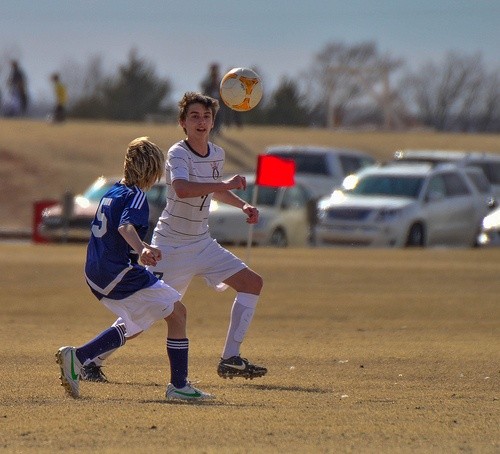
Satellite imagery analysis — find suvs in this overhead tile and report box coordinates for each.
[311,161,494,247]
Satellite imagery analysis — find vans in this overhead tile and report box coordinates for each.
[395,149,500,184]
[260,146,376,201]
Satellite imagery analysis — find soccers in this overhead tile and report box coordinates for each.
[220,67,263,112]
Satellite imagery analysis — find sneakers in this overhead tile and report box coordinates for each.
[216,353,268,381]
[79,361,110,383]
[165,380,215,400]
[54,346,84,399]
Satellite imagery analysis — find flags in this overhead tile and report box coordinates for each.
[255,154,296,187]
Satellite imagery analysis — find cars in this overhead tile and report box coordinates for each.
[207,175,311,248]
[476,197,499,246]
[36,176,169,244]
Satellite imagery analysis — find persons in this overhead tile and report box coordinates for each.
[8,58,66,124]
[54,91,268,402]
